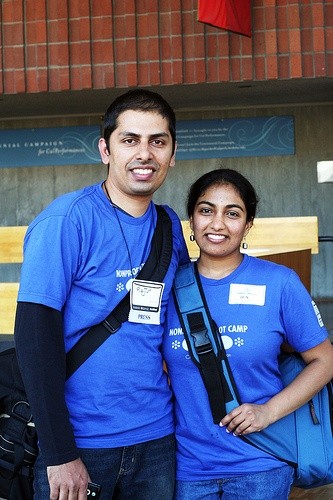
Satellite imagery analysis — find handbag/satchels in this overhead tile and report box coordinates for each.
[276,352,332,487]
[0,347,38,500]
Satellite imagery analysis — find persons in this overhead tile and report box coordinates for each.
[157,167,333,500]
[13,88,193,500]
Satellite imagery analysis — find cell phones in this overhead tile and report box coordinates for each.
[86,482,100,500]
